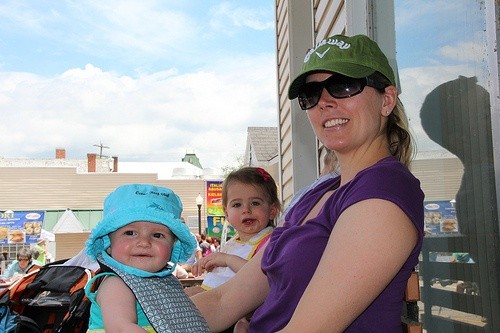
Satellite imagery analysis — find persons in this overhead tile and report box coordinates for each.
[183,147,341,333]
[187,34,426,333]
[181,217,220,267]
[7,248,39,278]
[30,248,45,266]
[85,184,211,333]
[191,166,283,290]
[35,238,47,264]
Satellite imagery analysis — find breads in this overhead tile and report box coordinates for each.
[0,222,24,242]
[442,219,456,231]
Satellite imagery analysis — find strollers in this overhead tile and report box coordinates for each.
[0,257,91,333]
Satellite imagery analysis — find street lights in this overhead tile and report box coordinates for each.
[196,193,204,235]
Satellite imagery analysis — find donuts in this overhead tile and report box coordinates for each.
[25,222,41,234]
[425,212,441,224]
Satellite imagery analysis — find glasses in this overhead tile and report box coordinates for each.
[297,73,383,111]
[17,258,29,262]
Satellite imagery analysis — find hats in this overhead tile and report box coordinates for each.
[85,184,197,278]
[287,34,396,101]
